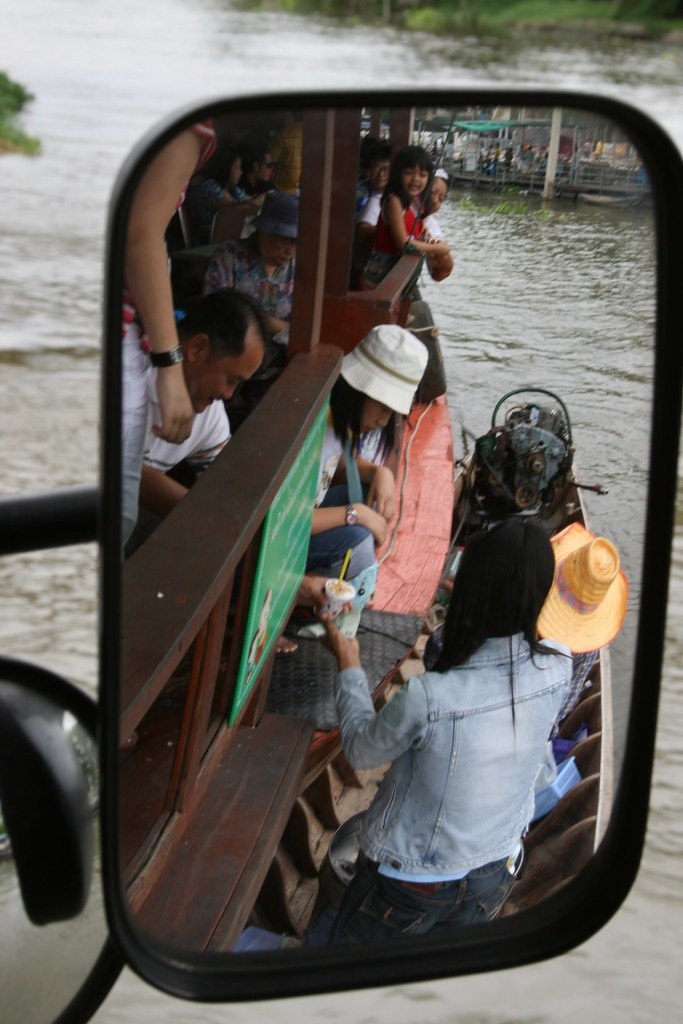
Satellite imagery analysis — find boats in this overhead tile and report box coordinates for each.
[234,389,618,953]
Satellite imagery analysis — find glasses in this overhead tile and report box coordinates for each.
[262,161,278,169]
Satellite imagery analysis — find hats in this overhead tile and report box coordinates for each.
[248,191,301,239]
[534,522,630,656]
[339,324,429,415]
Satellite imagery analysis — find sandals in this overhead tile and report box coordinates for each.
[276,639,299,658]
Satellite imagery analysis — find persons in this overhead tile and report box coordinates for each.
[121,102,626,954]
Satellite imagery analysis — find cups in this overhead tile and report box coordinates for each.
[314,578,356,622]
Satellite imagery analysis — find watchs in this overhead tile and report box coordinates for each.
[151,345,184,368]
[345,504,359,526]
